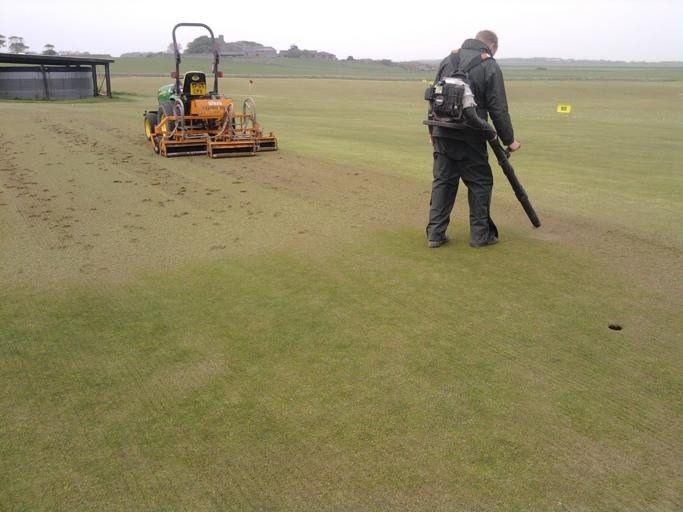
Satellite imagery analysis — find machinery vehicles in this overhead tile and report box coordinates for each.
[144,22,279,159]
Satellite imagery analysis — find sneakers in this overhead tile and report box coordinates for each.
[468,236,499,249]
[427,233,451,249]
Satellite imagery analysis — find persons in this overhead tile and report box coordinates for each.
[424,28,521,249]
